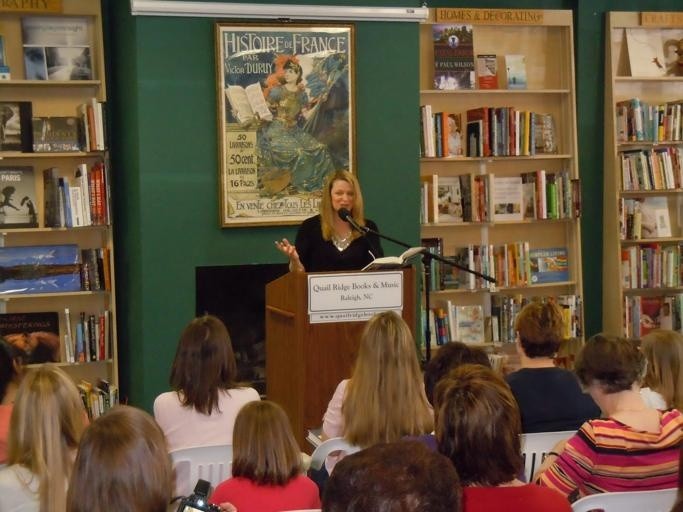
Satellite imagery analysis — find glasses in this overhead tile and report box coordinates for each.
[129,0,429,23]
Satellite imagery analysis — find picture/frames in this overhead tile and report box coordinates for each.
[264,264,417,447]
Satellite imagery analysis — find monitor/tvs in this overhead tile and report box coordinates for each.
[622,293,683,337]
[225,82,274,127]
[361,246,426,272]
[64,308,109,363]
[0,161,107,229]
[620,194,643,243]
[621,243,683,289]
[615,98,683,143]
[557,295,583,338]
[420,169,582,225]
[80,247,110,293]
[424,295,522,346]
[420,103,556,157]
[421,237,568,292]
[0,98,105,153]
[75,377,118,421]
[619,147,683,191]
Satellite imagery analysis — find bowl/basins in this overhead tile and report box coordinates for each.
[331,231,353,253]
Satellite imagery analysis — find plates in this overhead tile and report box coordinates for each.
[419,7,586,353]
[602,10,683,339]
[1,0,120,418]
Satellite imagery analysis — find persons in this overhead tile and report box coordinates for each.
[639,328,683,411]
[0,363,90,512]
[230,55,337,199]
[153,314,261,503]
[207,400,322,512]
[531,331,683,512]
[503,301,601,434]
[321,312,435,477]
[0,335,24,463]
[276,168,384,273]
[321,442,462,512]
[66,403,174,512]
[424,342,492,409]
[433,364,574,512]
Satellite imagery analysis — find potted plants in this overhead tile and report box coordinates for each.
[213,21,359,230]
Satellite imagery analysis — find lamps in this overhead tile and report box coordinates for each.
[569,487,682,511]
[308,437,361,477]
[518,429,580,485]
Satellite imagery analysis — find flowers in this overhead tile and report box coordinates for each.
[337,207,364,235]
